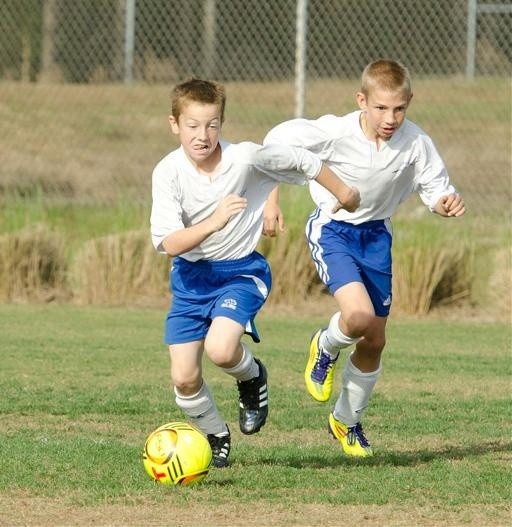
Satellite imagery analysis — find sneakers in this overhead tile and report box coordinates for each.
[328,411,373,458]
[207,425,230,469]
[237,357,268,436]
[305,327,340,403]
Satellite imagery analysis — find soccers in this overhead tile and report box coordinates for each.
[143,422,212,486]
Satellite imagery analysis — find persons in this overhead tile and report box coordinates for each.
[150,76,361,467]
[262,60,467,457]
